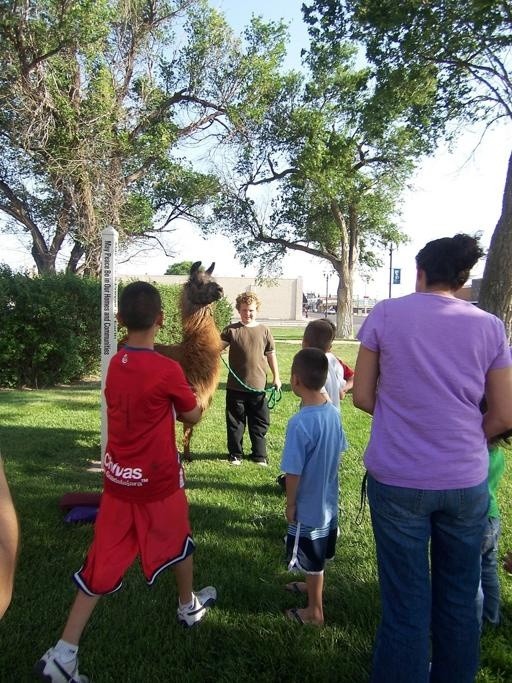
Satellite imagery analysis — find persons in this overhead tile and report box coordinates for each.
[35,282,217,683]
[0,457,20,620]
[219,291,282,468]
[280,319,353,626]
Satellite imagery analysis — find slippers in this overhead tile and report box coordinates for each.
[283,581,303,595]
[284,607,309,626]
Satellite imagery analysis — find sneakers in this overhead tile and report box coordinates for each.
[228,456,267,466]
[176,586,217,628]
[37,647,89,683]
